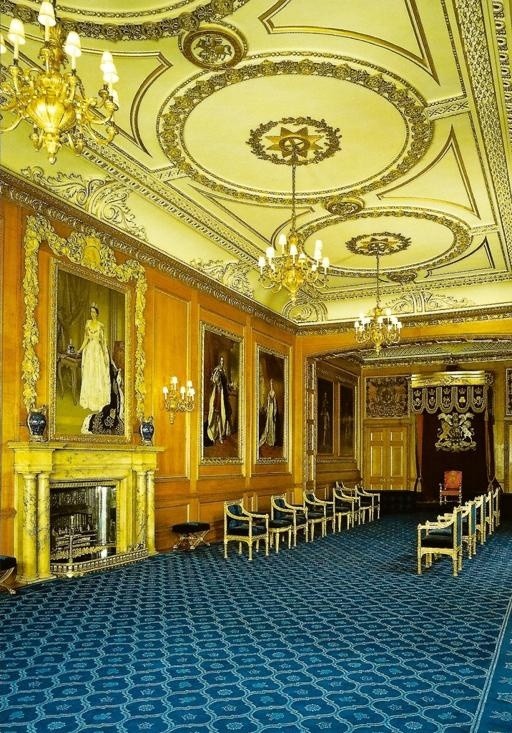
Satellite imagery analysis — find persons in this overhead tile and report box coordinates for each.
[77,302,118,412]
[260,378,279,448]
[206,354,237,444]
[320,391,332,447]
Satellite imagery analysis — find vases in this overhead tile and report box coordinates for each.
[25,408,47,442]
[139,421,154,446]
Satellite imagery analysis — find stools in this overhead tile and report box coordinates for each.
[173,522,211,550]
[0,555,17,595]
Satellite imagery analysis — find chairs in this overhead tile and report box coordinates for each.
[224,480,382,562]
[415,485,501,576]
[439,470,463,505]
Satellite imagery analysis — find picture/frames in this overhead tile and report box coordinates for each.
[505,368,512,415]
[316,376,356,457]
[254,341,289,465]
[48,256,133,444]
[197,320,248,465]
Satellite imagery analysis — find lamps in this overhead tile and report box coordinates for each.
[259,125,330,302]
[163,376,196,424]
[0,0,119,164]
[354,238,402,352]
